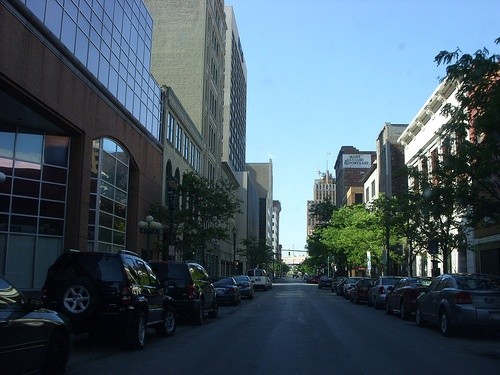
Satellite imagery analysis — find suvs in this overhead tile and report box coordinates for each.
[40,249,176,349]
[148,260,218,325]
[246,269,269,291]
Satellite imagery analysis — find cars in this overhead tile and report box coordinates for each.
[349,278,378,304]
[318,275,332,289]
[415,273,500,337]
[293,272,299,278]
[233,275,255,299]
[367,276,404,309]
[307,275,318,283]
[267,277,273,288]
[212,277,242,306]
[0,276,70,375]
[329,277,365,298]
[385,277,432,321]
